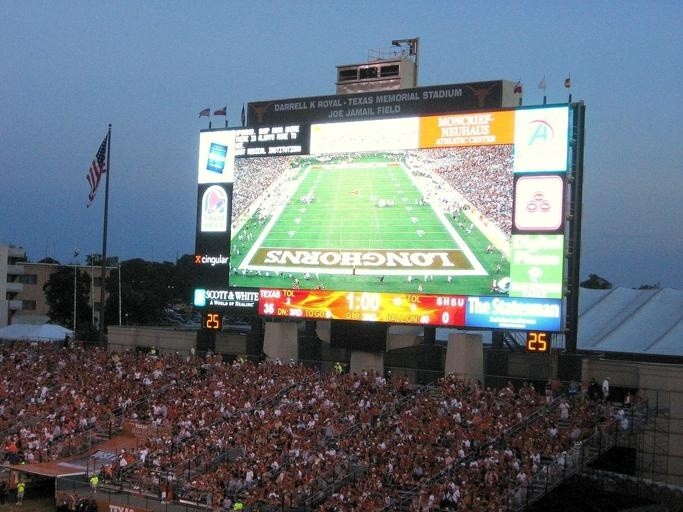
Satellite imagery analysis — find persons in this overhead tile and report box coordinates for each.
[229,144,514,296]
[1,340,632,512]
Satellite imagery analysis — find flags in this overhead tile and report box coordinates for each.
[565,78,570,88]
[213,107,226,115]
[539,79,544,89]
[85,129,109,210]
[513,83,523,94]
[240,106,245,125]
[198,109,210,118]
